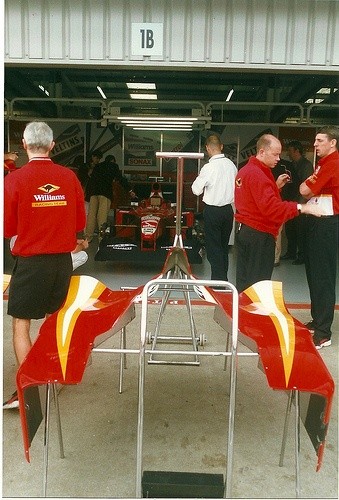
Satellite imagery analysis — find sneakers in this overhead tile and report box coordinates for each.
[312,336,332,349]
[304,320,316,334]
[3,391,20,409]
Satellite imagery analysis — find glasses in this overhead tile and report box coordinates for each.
[281,165,293,183]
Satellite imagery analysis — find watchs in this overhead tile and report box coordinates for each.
[297,204,302,214]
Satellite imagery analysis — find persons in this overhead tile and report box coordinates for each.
[76,150,103,236]
[86,155,135,242]
[299,128,339,350]
[3,121,86,410]
[191,135,238,290]
[234,134,320,295]
[272,141,314,267]
[4,141,88,271]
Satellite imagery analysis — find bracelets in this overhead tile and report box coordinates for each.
[129,190,132,192]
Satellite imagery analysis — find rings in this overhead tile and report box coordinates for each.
[282,176,285,179]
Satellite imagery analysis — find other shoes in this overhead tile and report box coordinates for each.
[281,254,295,260]
[292,260,304,265]
